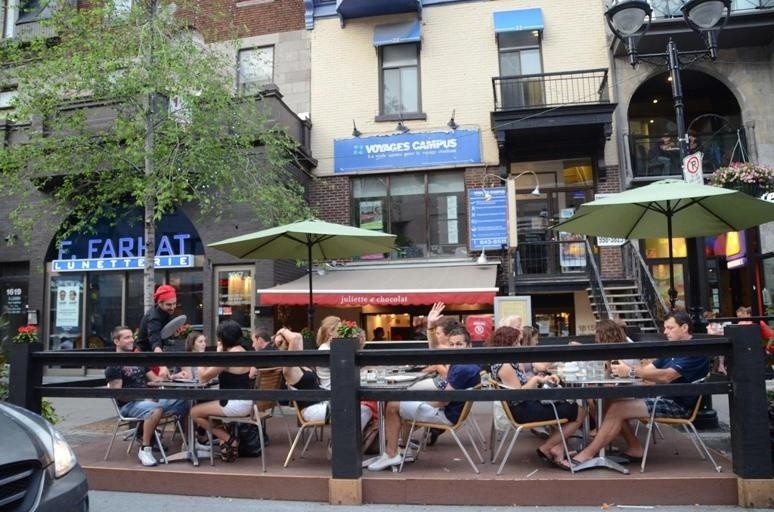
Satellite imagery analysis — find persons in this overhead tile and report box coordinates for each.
[646,130,705,175]
[704,307,774,375]
[105,287,274,471]
[489,310,712,471]
[275,298,481,471]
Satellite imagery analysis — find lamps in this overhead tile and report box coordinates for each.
[446,109,457,129]
[352,120,362,138]
[396,114,407,132]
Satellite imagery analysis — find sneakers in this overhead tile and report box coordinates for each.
[362,436,419,471]
[136,437,168,466]
[531,427,549,440]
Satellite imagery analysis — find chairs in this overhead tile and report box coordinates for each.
[103,383,189,466]
[206,366,293,472]
[488,376,570,475]
[395,384,487,474]
[633,371,722,474]
[283,382,331,468]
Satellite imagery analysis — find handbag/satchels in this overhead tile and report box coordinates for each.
[240,425,261,457]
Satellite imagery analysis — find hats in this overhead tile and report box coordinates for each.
[153,286,176,304]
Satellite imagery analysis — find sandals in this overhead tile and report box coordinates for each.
[219,435,239,463]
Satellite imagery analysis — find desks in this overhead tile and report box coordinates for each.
[558,371,643,476]
[147,379,219,467]
[359,365,430,472]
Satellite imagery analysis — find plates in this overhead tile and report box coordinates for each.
[159,314,187,342]
[386,371,426,381]
[173,378,198,384]
[561,365,581,373]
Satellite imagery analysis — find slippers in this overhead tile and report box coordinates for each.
[537,445,647,471]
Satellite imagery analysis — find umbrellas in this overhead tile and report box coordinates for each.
[205,219,397,329]
[546,179,774,310]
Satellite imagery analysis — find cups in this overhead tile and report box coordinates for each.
[360,365,404,385]
[585,359,605,381]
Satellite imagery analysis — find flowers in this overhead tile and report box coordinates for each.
[11,325,40,344]
[177,324,192,338]
[708,162,773,201]
[337,318,360,339]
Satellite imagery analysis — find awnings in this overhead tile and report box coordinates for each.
[257,287,499,305]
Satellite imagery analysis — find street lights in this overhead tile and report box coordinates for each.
[601,0,735,433]
[480,168,541,297]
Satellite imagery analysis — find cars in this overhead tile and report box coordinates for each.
[0,399,92,512]
